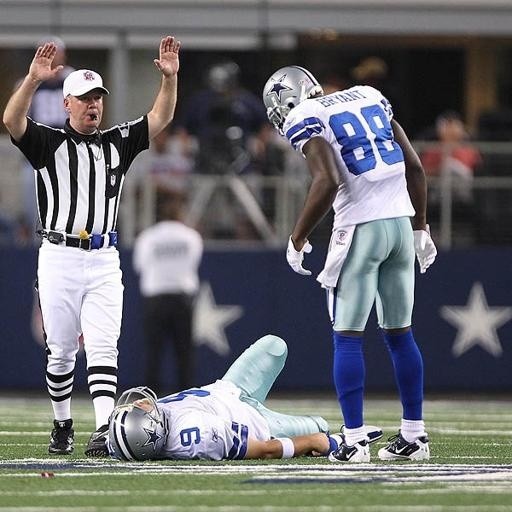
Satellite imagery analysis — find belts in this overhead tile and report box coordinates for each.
[42,230,117,252]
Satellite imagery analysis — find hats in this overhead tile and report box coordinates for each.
[63,69,110,99]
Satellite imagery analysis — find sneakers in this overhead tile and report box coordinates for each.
[326,436,370,463]
[47,418,74,455]
[377,428,431,461]
[84,424,109,459]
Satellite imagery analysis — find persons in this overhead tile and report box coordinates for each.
[263,65,439,463]
[2,35,181,459]
[133,190,203,397]
[0,29,511,244]
[108,334,383,461]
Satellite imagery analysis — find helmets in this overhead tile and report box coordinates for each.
[108,385,169,461]
[262,65,324,137]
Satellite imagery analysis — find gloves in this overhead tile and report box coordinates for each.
[285,234,314,277]
[413,222,438,274]
